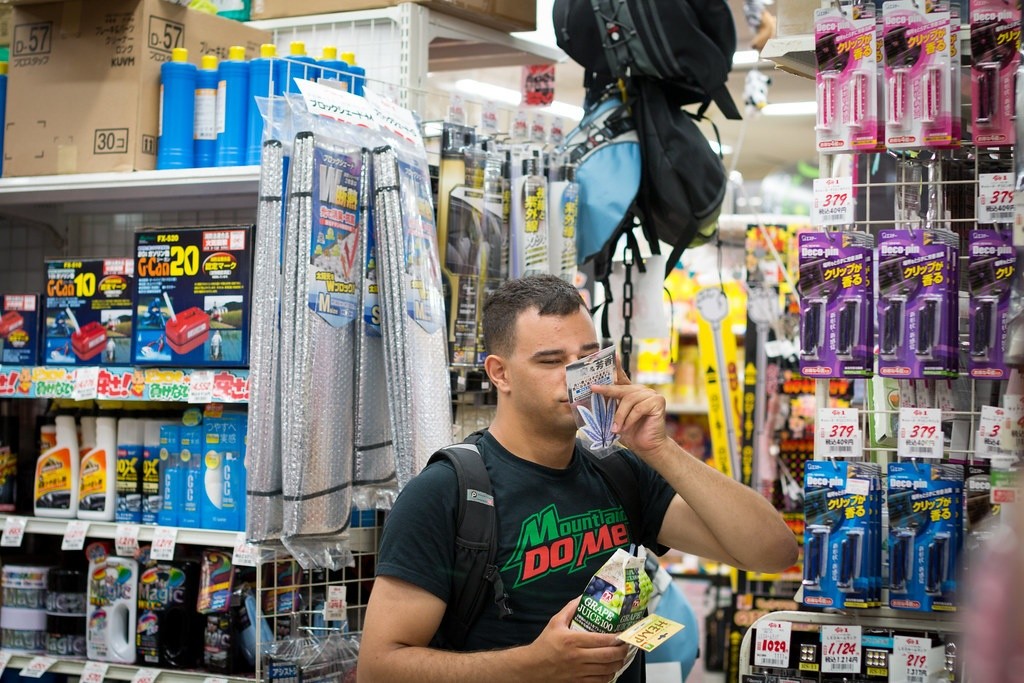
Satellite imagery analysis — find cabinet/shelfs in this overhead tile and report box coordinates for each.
[0,0,568,683]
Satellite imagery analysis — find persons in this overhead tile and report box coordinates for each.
[356,274,799,683]
[54,311,115,361]
[147,297,223,359]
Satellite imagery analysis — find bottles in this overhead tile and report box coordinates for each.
[32,398,180,525]
[157,39,364,170]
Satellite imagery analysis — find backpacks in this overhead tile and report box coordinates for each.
[553,0,744,279]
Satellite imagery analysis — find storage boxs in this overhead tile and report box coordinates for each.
[176,425,202,532]
[0,0,275,179]
[156,424,179,529]
[41,255,138,369]
[201,408,247,533]
[0,292,40,364]
[132,218,257,372]
